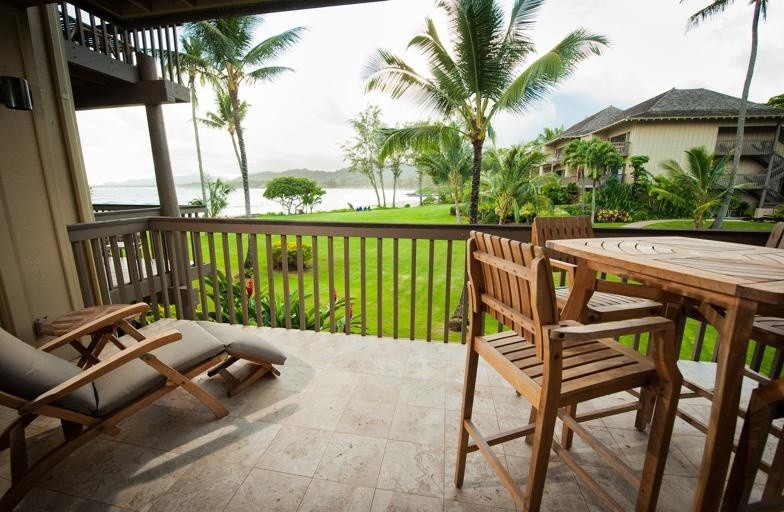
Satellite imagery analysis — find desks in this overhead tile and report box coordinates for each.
[521,233,784,511]
[42,303,139,371]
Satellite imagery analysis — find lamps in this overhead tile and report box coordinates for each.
[0,74,34,113]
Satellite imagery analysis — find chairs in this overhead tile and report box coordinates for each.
[455,228,685,512]
[719,378,784,511]
[1,301,286,512]
[658,220,784,499]
[513,214,665,454]
